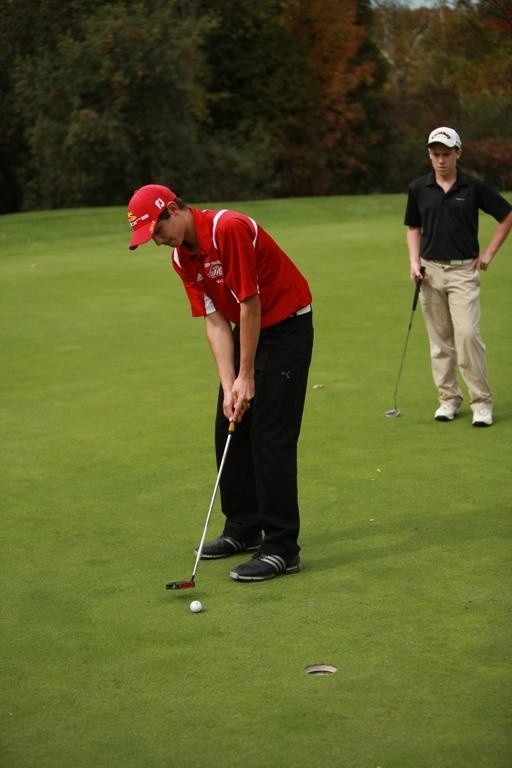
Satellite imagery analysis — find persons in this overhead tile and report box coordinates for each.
[122,182,313,584]
[401,123,512,429]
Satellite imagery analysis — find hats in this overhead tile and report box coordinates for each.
[128,184,176,250]
[427,127,462,149]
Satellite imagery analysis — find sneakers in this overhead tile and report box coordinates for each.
[229,552,300,581]
[434,404,460,420]
[473,405,493,426]
[194,533,263,558]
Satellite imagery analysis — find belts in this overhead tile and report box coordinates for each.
[434,259,474,265]
[289,304,311,317]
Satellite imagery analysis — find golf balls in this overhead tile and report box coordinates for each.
[190,601,202,611]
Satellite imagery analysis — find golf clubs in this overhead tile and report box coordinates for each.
[384,267,426,418]
[165,422,238,590]
